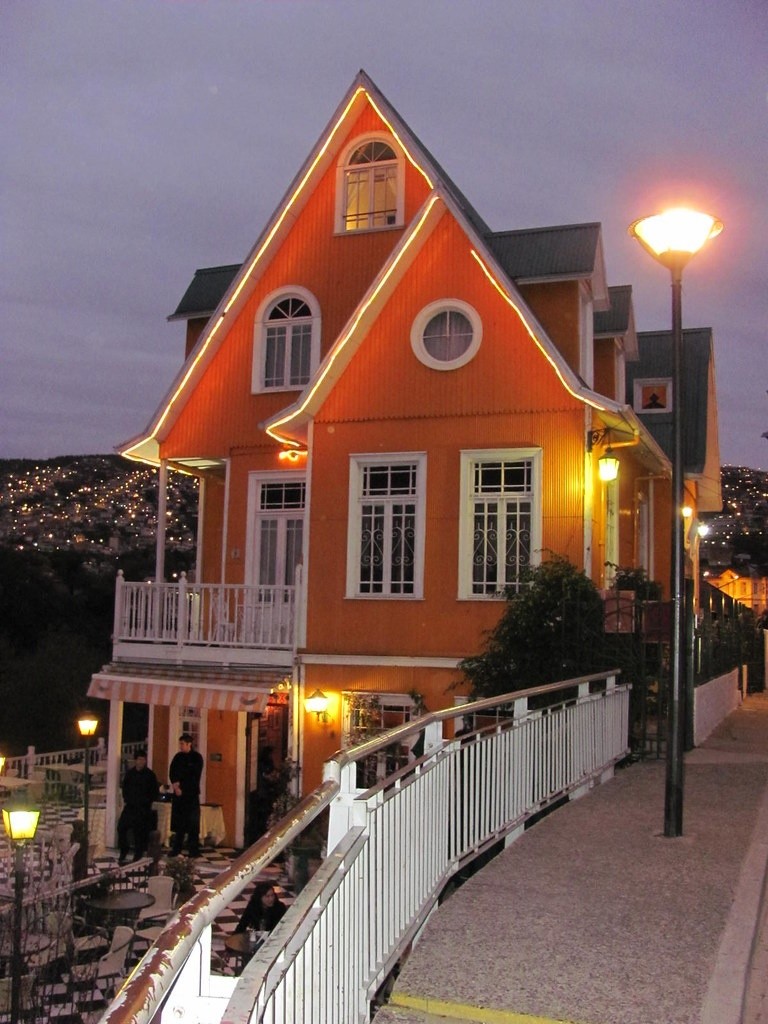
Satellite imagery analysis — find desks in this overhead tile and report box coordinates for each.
[235,602,286,645]
[146,801,226,850]
[85,891,155,927]
[225,931,268,956]
[0,932,52,968]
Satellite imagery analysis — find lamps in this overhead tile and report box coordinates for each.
[587,426,621,481]
[303,689,329,721]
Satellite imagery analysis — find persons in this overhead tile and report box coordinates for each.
[116,750,159,862]
[233,883,287,935]
[167,734,204,858]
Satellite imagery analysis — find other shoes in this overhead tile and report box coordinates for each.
[168,848,181,856]
[119,848,128,861]
[134,850,142,861]
[189,848,198,857]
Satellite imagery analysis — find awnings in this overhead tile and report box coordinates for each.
[87,664,292,714]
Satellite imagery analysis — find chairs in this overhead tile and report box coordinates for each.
[0,757,175,1024]
[210,594,237,647]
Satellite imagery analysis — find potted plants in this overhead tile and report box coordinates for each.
[269,791,318,893]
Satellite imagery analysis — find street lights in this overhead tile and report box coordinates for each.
[76,709,99,842]
[623,206,726,836]
[1,799,41,1024]
